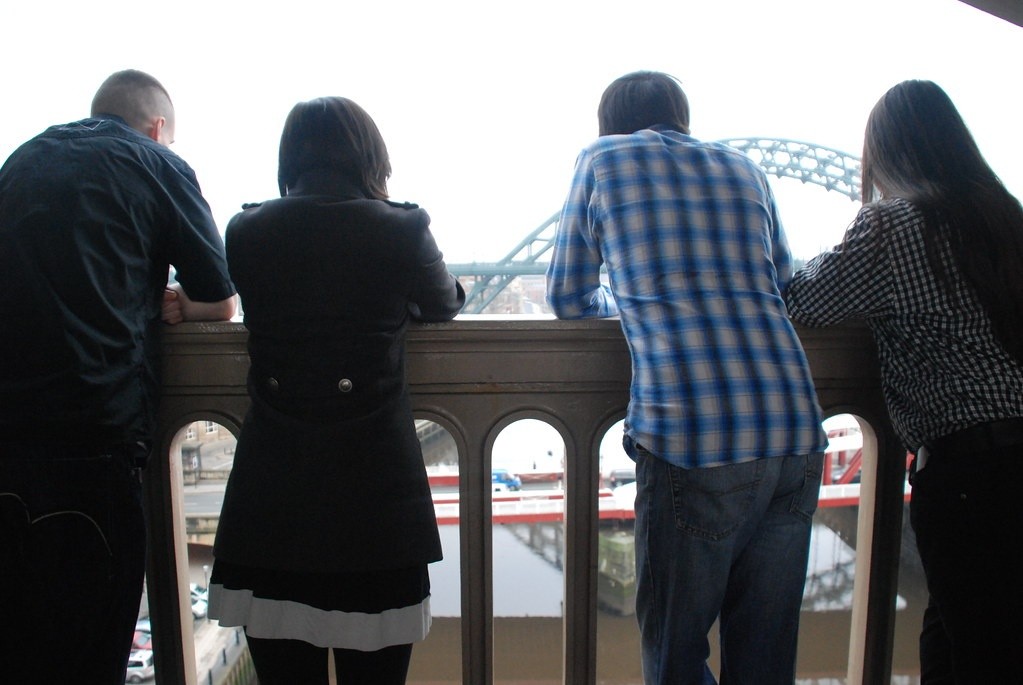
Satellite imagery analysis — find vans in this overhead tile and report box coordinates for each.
[491,469,522,490]
[121,649,157,683]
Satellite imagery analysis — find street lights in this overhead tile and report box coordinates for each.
[203,564,211,623]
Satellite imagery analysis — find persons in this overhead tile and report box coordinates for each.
[192,97,468,685]
[0,68,238,685]
[545,67,832,685]
[772,78,1023,685]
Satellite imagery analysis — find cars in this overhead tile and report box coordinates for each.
[188,582,210,602]
[828,466,862,487]
[187,596,208,621]
[491,482,511,494]
[130,631,154,652]
[135,615,156,632]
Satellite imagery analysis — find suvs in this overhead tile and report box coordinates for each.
[609,468,637,487]
